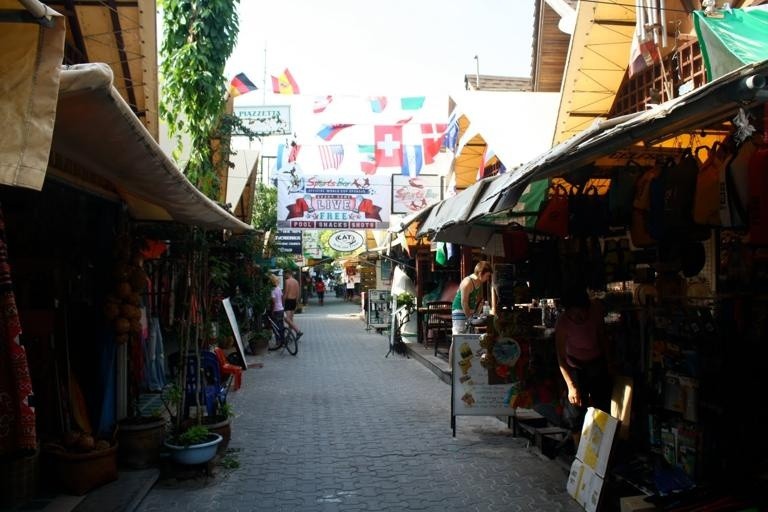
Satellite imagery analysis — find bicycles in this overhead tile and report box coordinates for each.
[254,307,299,357]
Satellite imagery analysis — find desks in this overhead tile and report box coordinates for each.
[433,315,452,357]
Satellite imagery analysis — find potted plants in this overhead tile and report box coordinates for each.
[249,327,274,356]
[159,224,250,457]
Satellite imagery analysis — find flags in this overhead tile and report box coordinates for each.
[231,67,448,179]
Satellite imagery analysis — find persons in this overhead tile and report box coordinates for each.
[447,260,492,374]
[305,273,356,306]
[554,289,603,451]
[281,269,303,341]
[271,277,284,349]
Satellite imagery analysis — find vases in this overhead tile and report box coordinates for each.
[113,415,168,470]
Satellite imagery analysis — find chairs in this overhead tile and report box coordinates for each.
[419,300,452,350]
